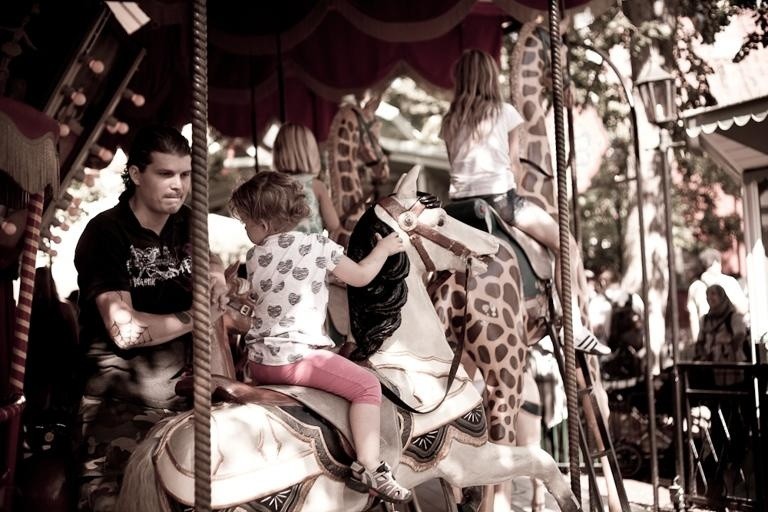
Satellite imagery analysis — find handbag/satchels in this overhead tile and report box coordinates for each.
[444,198,498,234]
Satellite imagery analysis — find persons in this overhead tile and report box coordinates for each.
[585,248,747,498]
[22,49,610,512]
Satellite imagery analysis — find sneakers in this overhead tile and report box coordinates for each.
[559,325,613,355]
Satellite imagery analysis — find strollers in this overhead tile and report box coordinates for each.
[613,372,679,478]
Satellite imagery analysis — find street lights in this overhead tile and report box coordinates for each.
[635,55,686,512]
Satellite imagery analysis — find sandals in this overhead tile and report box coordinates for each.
[344,460,414,505]
